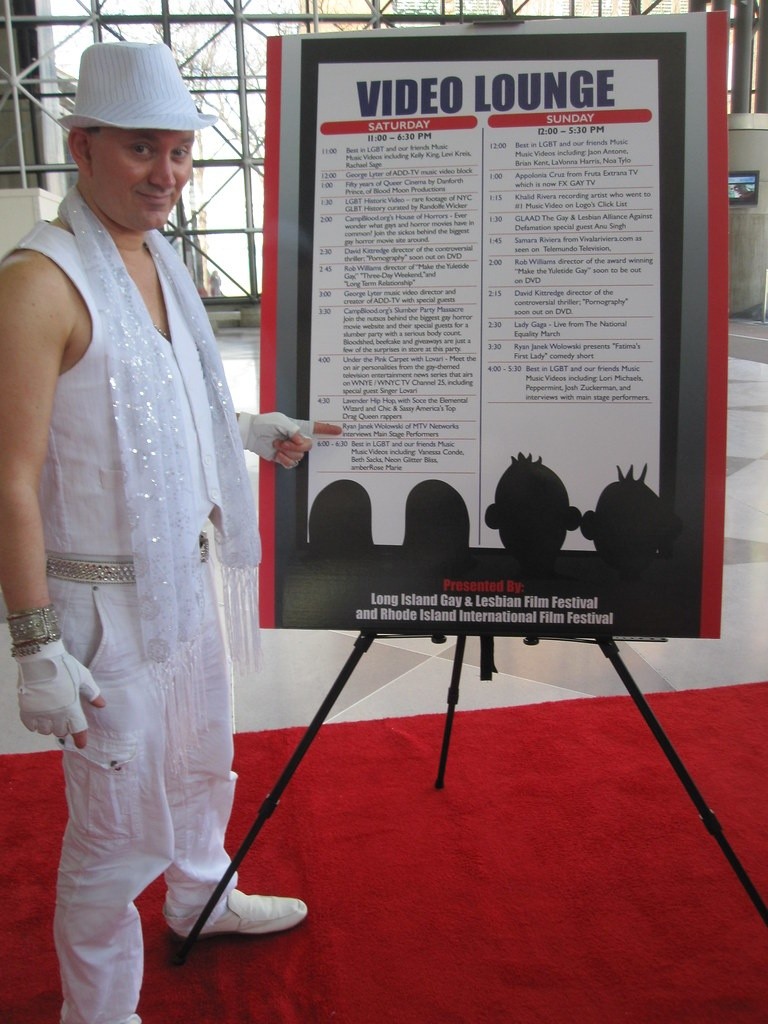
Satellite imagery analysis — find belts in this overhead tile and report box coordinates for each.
[47,534,209,583]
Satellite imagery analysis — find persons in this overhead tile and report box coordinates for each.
[0,42,343,1024]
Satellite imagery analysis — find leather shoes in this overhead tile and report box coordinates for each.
[168,890,308,947]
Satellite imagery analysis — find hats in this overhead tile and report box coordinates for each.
[57,41,217,130]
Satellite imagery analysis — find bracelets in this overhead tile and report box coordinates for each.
[6,604,62,657]
